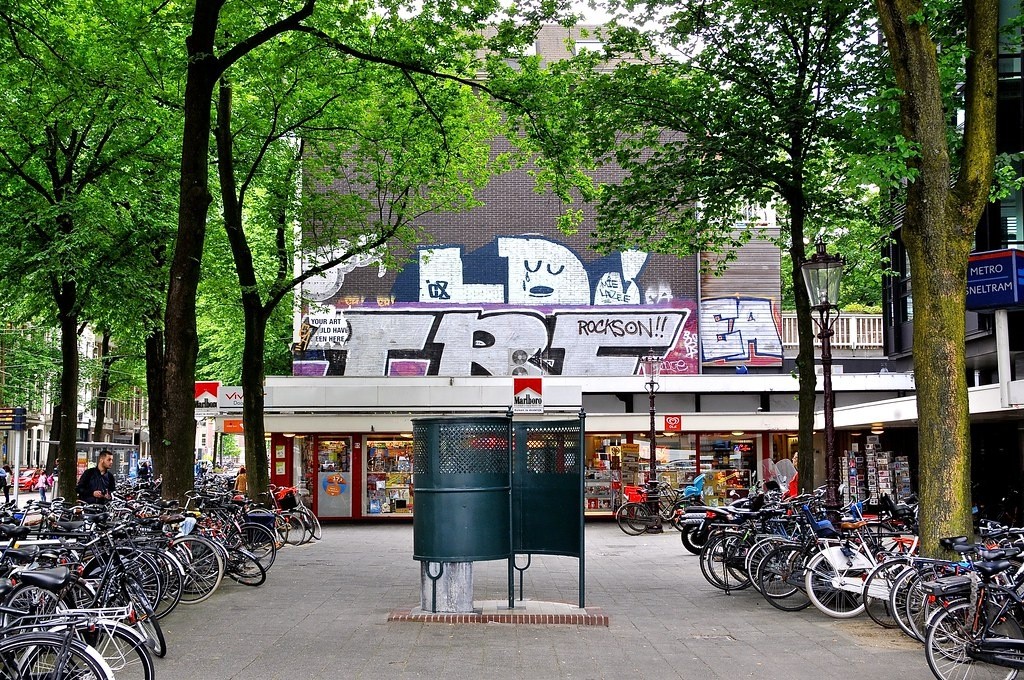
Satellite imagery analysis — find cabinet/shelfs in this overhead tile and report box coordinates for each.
[584,469,613,511]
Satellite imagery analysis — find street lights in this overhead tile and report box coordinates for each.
[638,349,665,533]
[800,237,850,531]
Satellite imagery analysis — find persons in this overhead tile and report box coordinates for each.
[769,466,783,483]
[75,451,115,504]
[195,460,209,476]
[0,466,12,503]
[267,455,270,467]
[234,465,246,491]
[52,459,60,476]
[138,457,151,478]
[789,451,798,496]
[35,470,47,502]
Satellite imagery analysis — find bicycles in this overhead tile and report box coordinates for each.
[0,473,323,680]
[615,471,1024,680]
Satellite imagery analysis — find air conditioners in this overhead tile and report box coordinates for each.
[508,347,543,376]
[814,364,843,376]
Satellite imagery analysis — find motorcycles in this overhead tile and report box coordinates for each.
[674,458,799,555]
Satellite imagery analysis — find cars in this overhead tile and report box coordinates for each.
[8,468,45,492]
